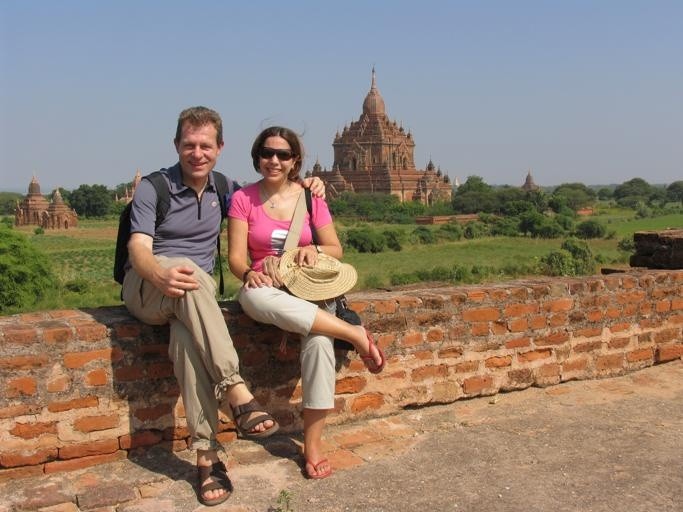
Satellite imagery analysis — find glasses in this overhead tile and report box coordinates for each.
[259,146,295,162]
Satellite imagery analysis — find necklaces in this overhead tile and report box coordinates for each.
[262,183,287,208]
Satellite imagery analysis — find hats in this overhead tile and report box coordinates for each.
[279,247,358,301]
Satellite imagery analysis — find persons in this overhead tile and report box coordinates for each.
[114,107,327,506]
[225,126,384,480]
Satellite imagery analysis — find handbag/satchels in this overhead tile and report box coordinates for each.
[263,255,293,287]
[332,308,362,351]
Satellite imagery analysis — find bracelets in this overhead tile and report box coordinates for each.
[242,267,252,285]
[315,244,322,254]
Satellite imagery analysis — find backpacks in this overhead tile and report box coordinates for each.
[113,170,230,286]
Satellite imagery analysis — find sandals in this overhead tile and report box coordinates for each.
[196,456,232,506]
[228,394,280,440]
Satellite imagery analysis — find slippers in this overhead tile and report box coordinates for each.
[354,328,385,374]
[305,451,331,479]
